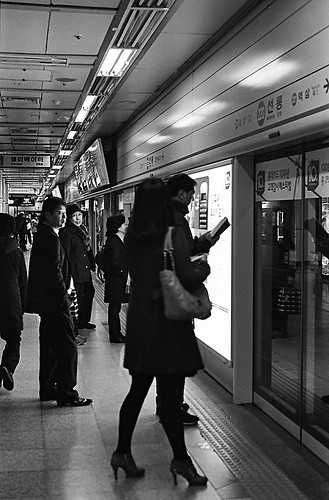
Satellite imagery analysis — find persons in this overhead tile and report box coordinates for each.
[0,213,28,391]
[30,212,40,235]
[56,204,97,331]
[154,173,230,426]
[93,212,129,344]
[24,214,33,246]
[62,274,87,347]
[109,177,209,489]
[27,196,93,408]
[17,212,29,252]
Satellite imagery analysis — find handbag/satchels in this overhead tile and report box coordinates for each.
[161,224,212,320]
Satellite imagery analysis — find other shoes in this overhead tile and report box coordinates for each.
[110,336,126,343]
[40,393,58,401]
[57,398,93,406]
[87,323,96,329]
[184,402,189,412]
[75,335,87,345]
[0,365,15,391]
[183,413,199,426]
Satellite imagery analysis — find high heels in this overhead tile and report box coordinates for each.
[110,450,145,480]
[169,454,208,488]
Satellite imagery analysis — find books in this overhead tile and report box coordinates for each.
[210,216,230,238]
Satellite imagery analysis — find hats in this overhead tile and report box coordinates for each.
[66,203,87,220]
